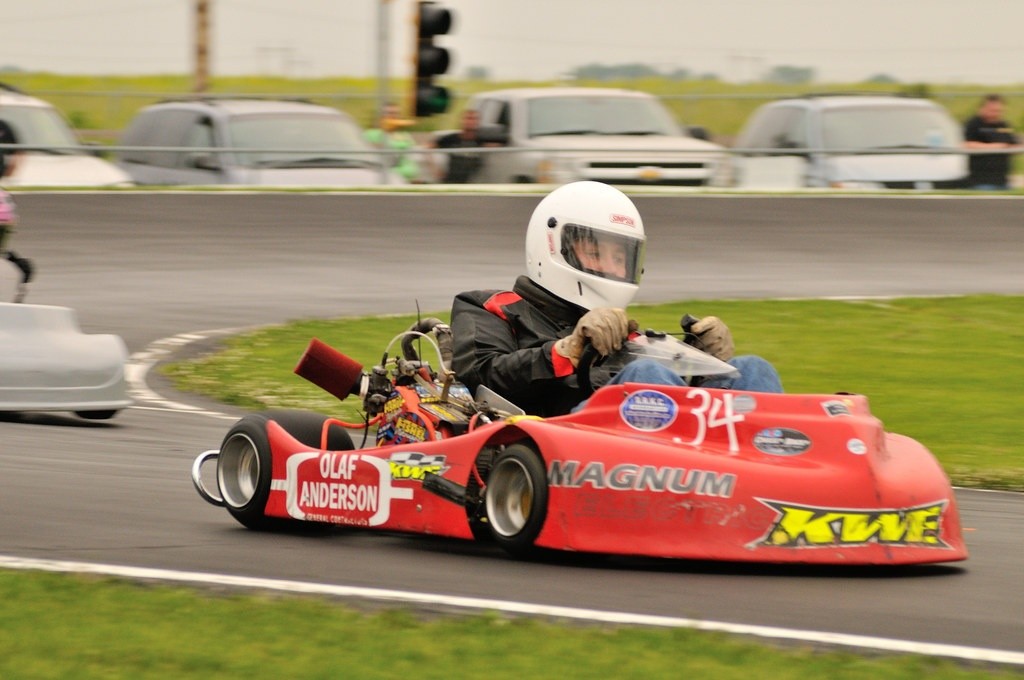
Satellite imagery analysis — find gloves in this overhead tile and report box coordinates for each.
[555,307,639,371]
[680,313,735,363]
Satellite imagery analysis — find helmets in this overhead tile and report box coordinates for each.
[524,180,647,313]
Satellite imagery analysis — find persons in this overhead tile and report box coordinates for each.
[0,119,33,304]
[450,180,783,418]
[964,95,1024,192]
[361,102,509,184]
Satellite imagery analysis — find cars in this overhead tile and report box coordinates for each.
[727,94,973,194]
[0,82,130,191]
[423,85,737,191]
[115,90,410,189]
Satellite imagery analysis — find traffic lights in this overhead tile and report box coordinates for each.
[408,1,452,116]
